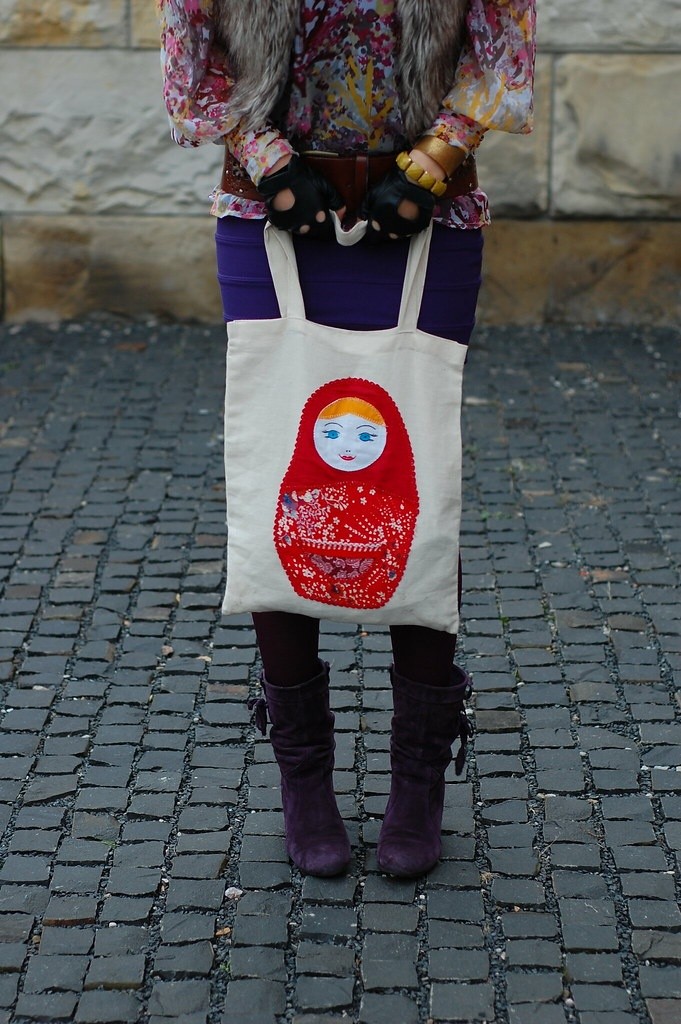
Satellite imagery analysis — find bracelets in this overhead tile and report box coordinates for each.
[412,135,465,179]
[395,150,447,197]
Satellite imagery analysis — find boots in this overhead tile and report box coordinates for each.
[375,660,474,882]
[248,657,351,881]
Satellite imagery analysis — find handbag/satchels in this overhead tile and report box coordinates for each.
[221,218,469,634]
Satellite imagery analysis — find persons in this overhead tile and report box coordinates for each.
[159,0,535,881]
